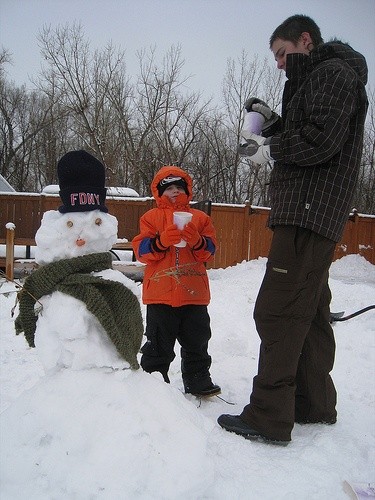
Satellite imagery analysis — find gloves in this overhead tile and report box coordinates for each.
[183,221,202,249]
[156,224,183,251]
[243,97,280,131]
[237,132,276,166]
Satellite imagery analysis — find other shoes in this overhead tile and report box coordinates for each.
[184,380,235,410]
[294,415,337,425]
[218,414,292,447]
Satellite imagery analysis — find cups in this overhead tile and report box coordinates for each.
[241,111,266,147]
[172,211,193,248]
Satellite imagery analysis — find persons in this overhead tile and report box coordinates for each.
[217,15,369,447]
[130,166,221,396]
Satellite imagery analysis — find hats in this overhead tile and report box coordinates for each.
[156,176,189,197]
[57,150,109,213]
